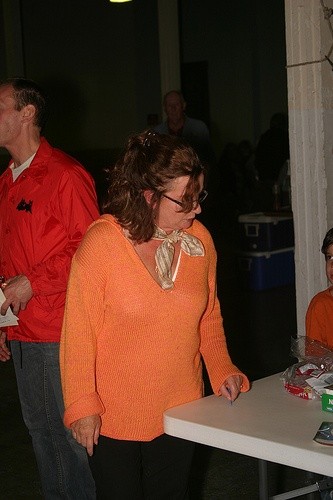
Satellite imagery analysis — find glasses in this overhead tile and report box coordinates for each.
[161,190,208,210]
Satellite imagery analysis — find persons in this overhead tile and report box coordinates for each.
[0,77,101,500]
[153,90,210,228]
[305,228,333,500]
[59,131,251,500]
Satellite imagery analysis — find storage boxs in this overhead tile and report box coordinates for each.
[236,212,295,291]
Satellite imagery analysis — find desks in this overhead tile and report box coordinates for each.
[163,368,333,500]
